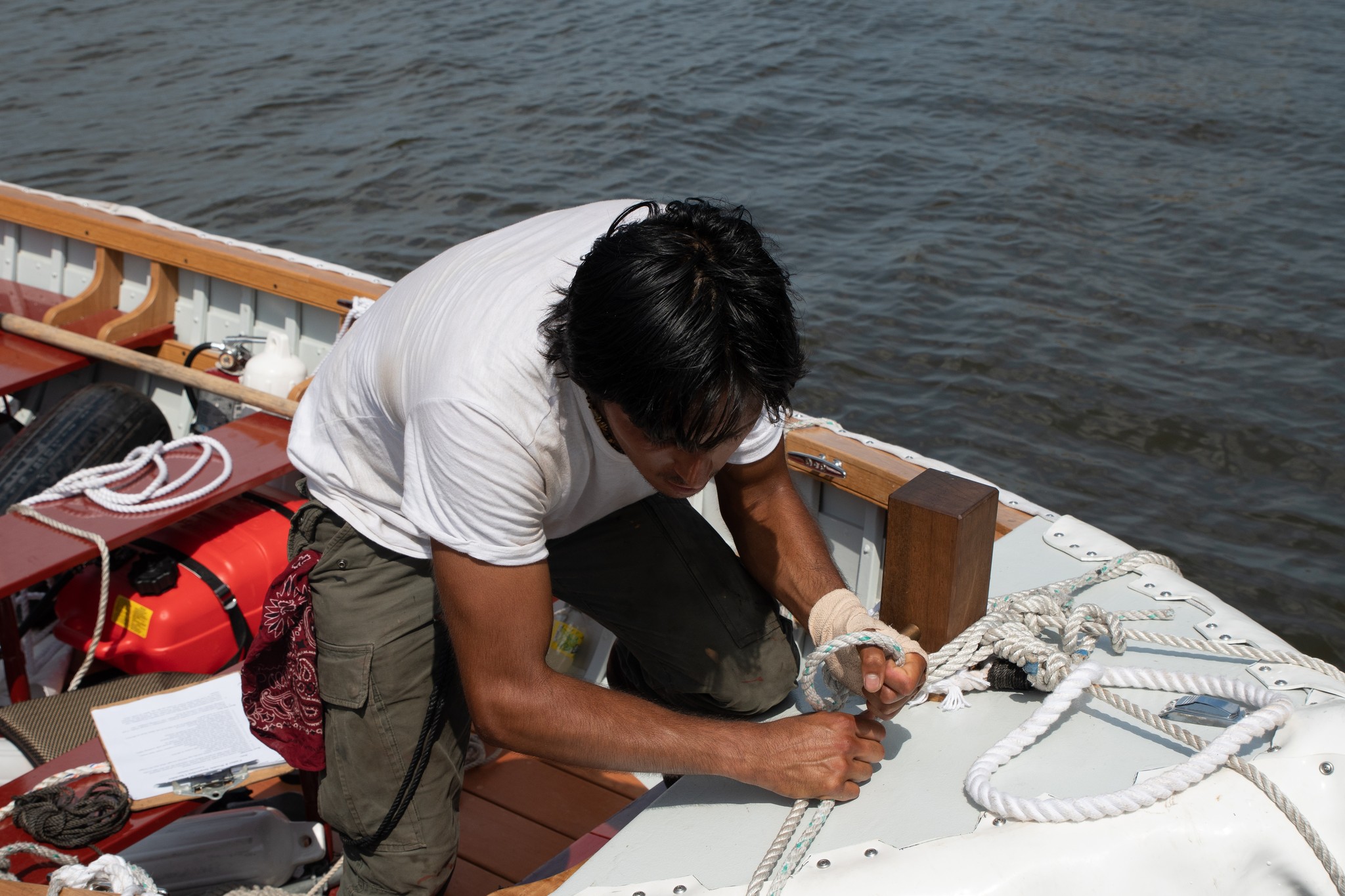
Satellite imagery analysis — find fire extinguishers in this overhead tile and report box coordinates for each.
[181,332,269,434]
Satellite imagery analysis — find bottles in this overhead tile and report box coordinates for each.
[545,604,586,675]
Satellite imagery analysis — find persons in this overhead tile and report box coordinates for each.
[276,194,932,896]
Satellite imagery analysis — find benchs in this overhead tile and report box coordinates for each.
[0,308,174,398]
[0,408,297,701]
